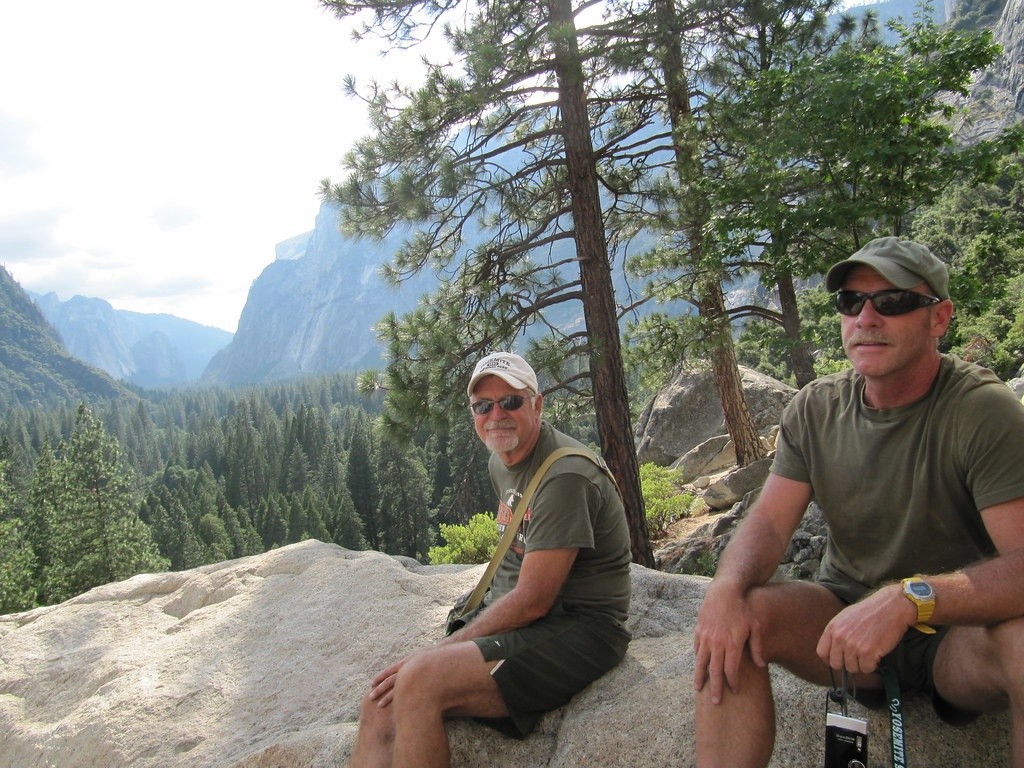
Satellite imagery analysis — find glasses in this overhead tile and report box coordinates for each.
[836,290,941,316]
[469,395,537,414]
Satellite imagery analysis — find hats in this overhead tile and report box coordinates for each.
[825,238,950,302]
[467,352,538,398]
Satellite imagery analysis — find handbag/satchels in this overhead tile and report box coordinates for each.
[445,589,493,639]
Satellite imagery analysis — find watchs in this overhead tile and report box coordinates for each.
[900,578,937,634]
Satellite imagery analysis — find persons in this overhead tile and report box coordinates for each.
[692,237,1024,768]
[350,352,633,768]
